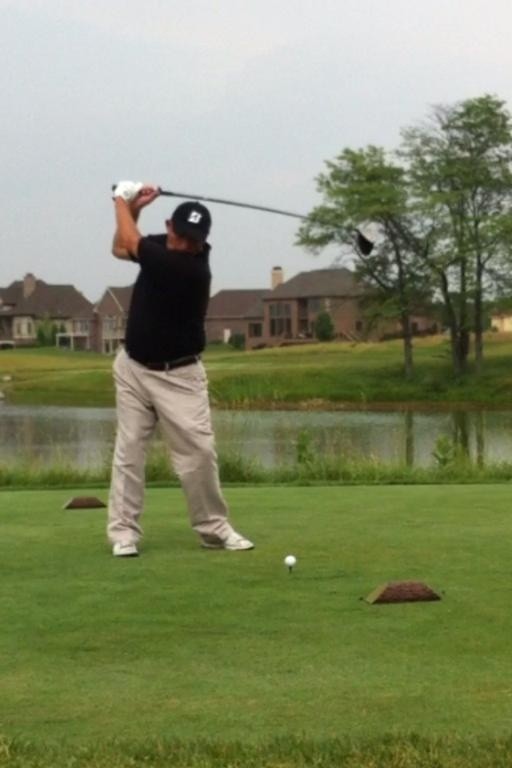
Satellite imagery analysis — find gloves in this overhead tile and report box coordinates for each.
[112,179,143,205]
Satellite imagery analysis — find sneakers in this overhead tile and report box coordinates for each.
[201,531,254,551]
[112,540,139,557]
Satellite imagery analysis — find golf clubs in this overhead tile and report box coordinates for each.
[112,184,374,256]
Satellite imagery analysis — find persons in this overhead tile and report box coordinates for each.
[103,177,259,560]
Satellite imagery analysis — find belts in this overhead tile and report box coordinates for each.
[124,345,200,371]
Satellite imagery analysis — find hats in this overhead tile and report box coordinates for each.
[171,201,210,240]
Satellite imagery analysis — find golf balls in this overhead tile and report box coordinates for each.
[285,555,296,566]
